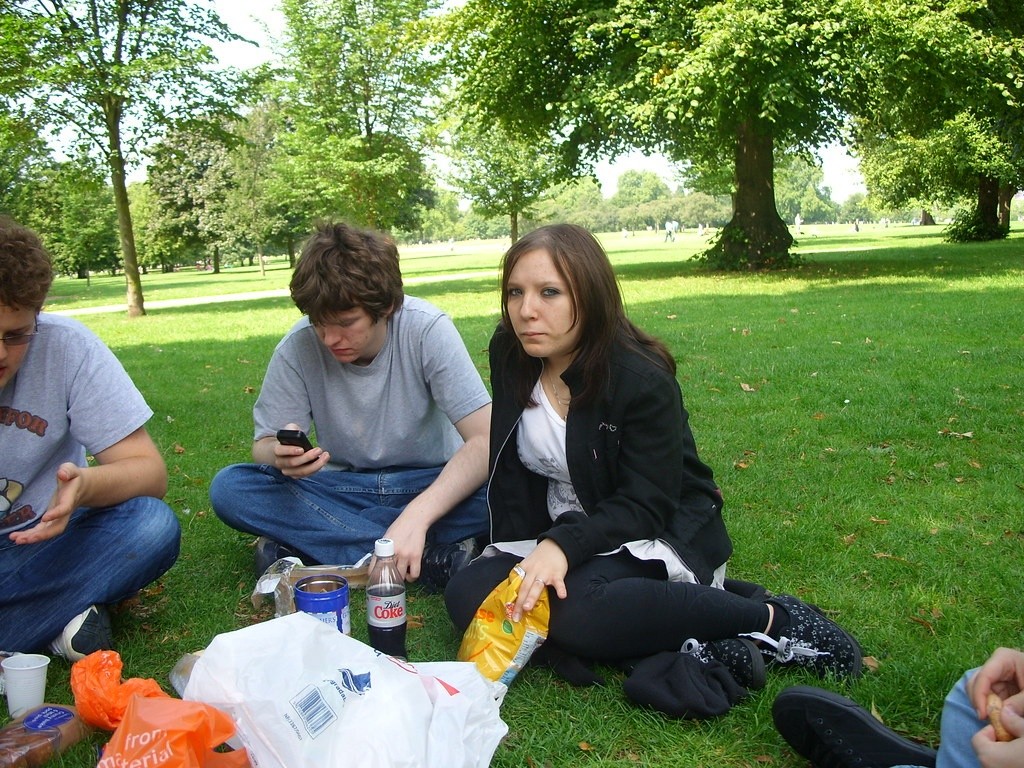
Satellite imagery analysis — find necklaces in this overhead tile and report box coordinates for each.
[547,363,567,421]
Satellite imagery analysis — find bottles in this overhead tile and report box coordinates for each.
[366,539,408,662]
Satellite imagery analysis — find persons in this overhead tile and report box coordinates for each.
[795,214,801,234]
[208,221,492,587]
[443,224,863,687]
[0,215,181,700]
[772,647,1024,768]
[664,219,679,241]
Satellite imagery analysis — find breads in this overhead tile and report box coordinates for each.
[0,702,95,768]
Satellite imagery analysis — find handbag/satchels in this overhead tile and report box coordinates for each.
[183,610,509,768]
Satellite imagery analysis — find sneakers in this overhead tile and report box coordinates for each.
[49,603,113,661]
[422,538,479,596]
[767,592,862,681]
[255,536,318,576]
[772,685,938,768]
[682,636,765,690]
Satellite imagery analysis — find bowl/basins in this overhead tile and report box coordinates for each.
[291,564,368,584]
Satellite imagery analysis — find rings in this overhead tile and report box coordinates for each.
[535,578,546,588]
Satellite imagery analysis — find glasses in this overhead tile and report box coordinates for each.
[1,315,38,346]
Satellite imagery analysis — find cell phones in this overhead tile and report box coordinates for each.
[277,430,320,465]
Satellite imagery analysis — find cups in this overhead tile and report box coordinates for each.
[294,574,350,637]
[1,654,50,719]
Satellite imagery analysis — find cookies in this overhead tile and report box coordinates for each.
[986,694,1015,741]
